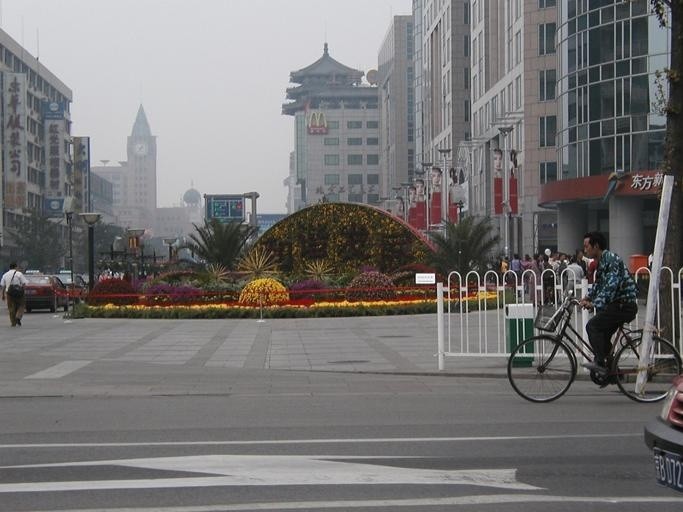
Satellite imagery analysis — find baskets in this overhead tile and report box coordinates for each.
[535,306,564,332]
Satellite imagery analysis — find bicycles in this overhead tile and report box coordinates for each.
[507,290,683,404]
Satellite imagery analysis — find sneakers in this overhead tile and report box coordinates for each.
[580,362,610,378]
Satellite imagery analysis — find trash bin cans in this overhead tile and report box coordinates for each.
[629,255,647,273]
[505,303,534,367]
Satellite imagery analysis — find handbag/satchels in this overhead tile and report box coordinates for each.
[8,284,24,298]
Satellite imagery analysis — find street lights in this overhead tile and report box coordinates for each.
[78,212,102,294]
[129,227,146,281]
[497,126,515,258]
[163,237,176,260]
[438,148,451,238]
[381,160,433,241]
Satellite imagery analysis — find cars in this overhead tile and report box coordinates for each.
[48,271,87,303]
[643,374,683,491]
[20,270,68,312]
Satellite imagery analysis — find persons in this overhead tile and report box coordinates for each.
[648,249,654,271]
[495,247,598,306]
[395,167,442,218]
[493,148,504,179]
[578,232,640,389]
[0,262,27,327]
